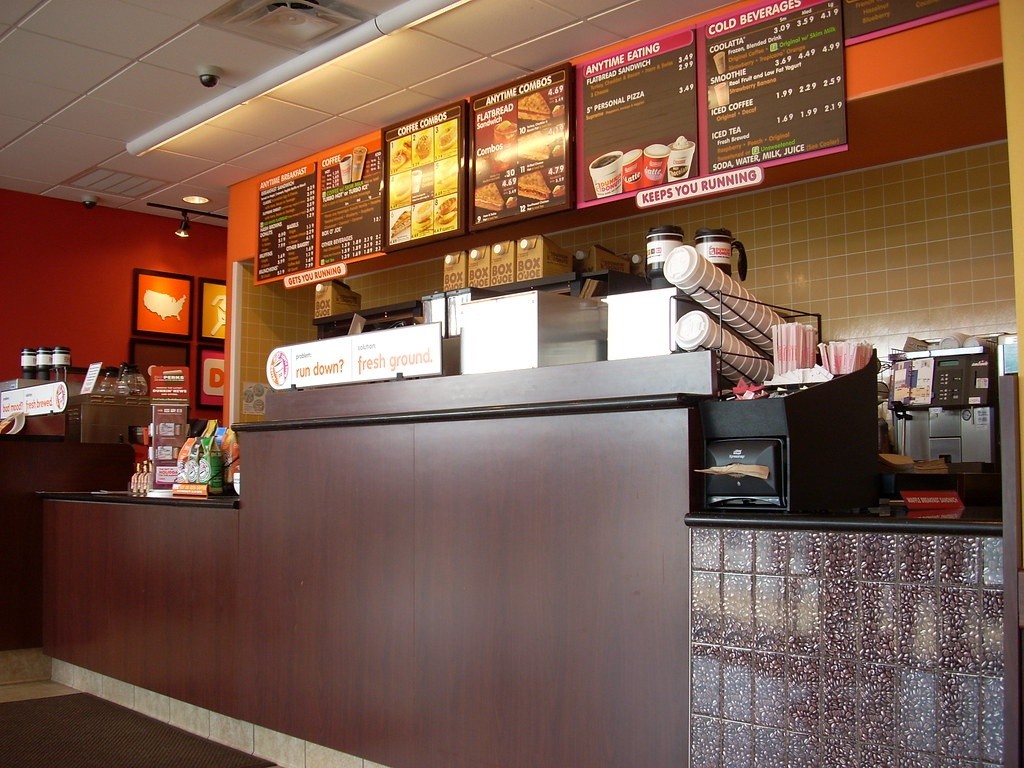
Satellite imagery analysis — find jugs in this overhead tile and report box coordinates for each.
[692,228,747,282]
[115,362,148,396]
[92,366,118,395]
[644,224,685,278]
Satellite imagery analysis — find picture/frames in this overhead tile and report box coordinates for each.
[127,267,227,411]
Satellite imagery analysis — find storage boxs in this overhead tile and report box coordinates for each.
[443,234,646,293]
[314,281,362,319]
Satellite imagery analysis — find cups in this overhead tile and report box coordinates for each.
[411,169,423,193]
[589,150,623,198]
[667,140,696,183]
[339,154,353,185]
[494,123,518,144]
[714,82,729,106]
[274,360,284,385]
[661,245,789,388]
[353,146,368,182]
[58,391,64,409]
[622,148,643,192]
[644,143,671,187]
[713,52,726,74]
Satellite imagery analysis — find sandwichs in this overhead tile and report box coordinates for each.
[517,92,552,120]
[517,171,551,201]
[391,210,411,238]
[474,182,505,212]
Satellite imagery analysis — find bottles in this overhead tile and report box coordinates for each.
[128,459,153,494]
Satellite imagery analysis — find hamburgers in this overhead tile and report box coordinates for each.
[436,197,456,224]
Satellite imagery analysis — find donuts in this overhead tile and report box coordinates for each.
[390,140,412,171]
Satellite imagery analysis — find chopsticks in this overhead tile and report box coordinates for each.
[770,322,875,375]
